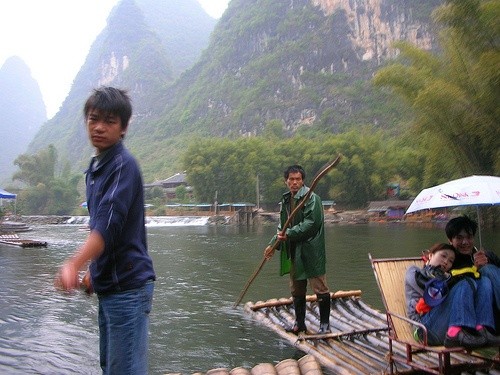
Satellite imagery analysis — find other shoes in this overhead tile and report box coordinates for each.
[443,330,486,348]
[468,327,500,346]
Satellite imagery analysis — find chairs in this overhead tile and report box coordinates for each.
[366,253,467,375]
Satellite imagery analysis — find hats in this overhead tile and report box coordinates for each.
[422,278,451,306]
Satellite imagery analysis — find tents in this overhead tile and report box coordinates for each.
[0,189,17,222]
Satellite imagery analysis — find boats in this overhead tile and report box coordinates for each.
[1,230,48,248]
[245,289,500,375]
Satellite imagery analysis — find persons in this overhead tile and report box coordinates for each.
[53,88,156,375]
[404,243,491,348]
[265,166,331,334]
[445,215,500,346]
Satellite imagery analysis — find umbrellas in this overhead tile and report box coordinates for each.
[404,175,500,251]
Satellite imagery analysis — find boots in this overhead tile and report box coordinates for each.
[284,295,306,334]
[317,293,331,335]
